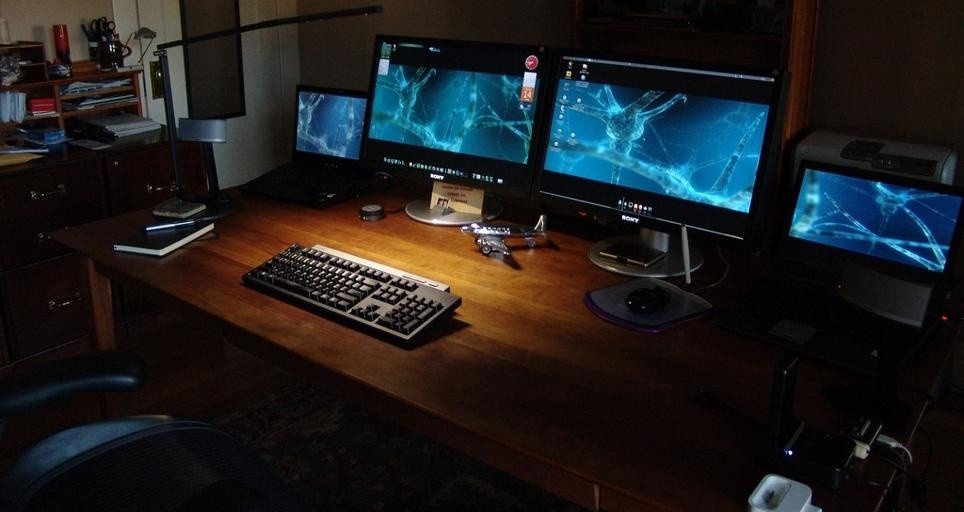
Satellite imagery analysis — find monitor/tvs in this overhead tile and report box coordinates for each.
[530,48,786,279]
[362,33,556,226]
[783,159,964,290]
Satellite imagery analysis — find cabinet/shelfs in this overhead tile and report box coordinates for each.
[4,66,143,152]
[5,138,206,378]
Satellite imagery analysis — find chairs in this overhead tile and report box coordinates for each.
[0,349,313,512]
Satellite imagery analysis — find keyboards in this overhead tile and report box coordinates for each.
[240,241,462,345]
[711,298,880,375]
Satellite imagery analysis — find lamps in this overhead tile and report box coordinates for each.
[176,117,231,205]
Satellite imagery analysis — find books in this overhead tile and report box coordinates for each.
[112,217,215,257]
[151,195,207,220]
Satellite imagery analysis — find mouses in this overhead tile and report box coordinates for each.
[623,285,673,315]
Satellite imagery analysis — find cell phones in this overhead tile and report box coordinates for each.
[599,240,666,267]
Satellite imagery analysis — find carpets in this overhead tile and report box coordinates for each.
[207,379,583,511]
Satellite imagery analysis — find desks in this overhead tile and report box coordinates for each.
[41,171,963,511]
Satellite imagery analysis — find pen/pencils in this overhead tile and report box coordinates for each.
[143,217,200,232]
[81,24,90,42]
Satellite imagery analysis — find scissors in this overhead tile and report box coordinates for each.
[91,16,115,40]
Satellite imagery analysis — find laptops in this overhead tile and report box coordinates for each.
[238,82,371,209]
